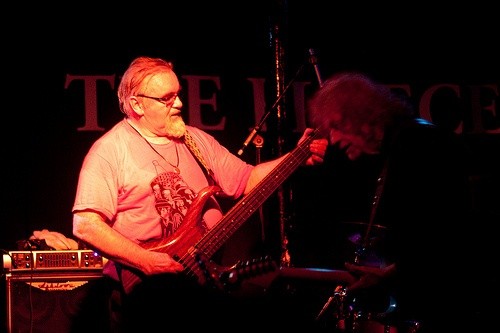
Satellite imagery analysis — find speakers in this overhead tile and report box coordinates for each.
[4,274,105,333]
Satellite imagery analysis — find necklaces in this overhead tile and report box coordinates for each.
[126,116,180,174]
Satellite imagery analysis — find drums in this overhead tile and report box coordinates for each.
[336,314,423,333]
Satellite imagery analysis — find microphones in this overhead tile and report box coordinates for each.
[309,49,322,87]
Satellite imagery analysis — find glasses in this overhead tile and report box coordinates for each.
[134,86,184,106]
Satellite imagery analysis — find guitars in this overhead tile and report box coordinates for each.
[120,128,327,298]
[232,223,391,291]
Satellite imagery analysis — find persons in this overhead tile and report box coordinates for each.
[307,71,500,333]
[73,55,328,333]
